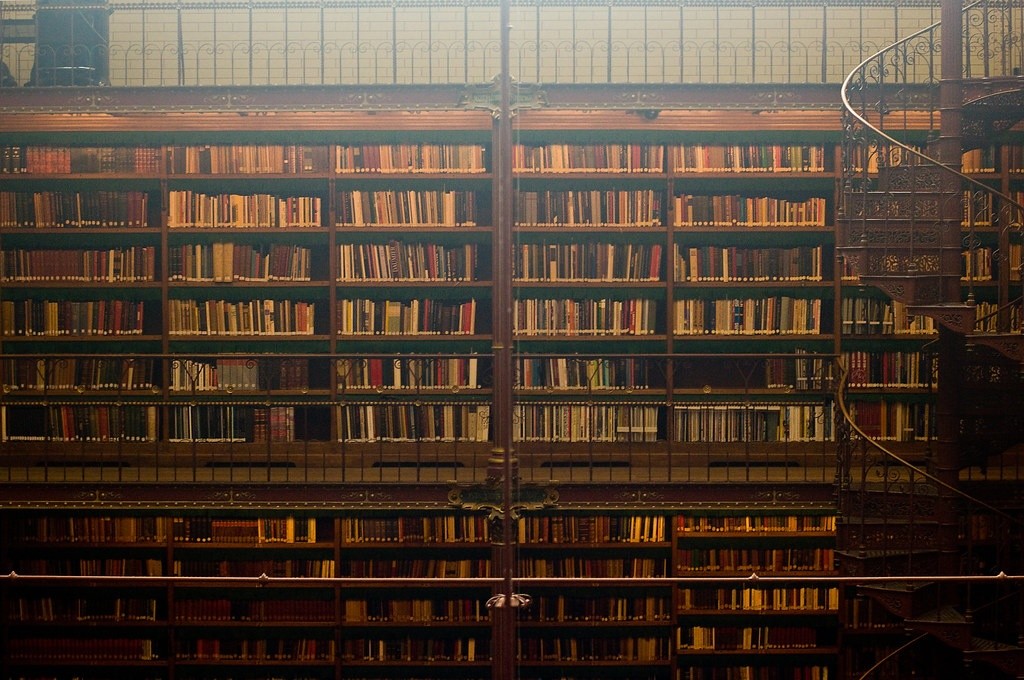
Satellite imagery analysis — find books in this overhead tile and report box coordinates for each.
[0,513,857,680]
[0,141,1024,449]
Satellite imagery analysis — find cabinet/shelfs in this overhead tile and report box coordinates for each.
[1,107,1024,680]
